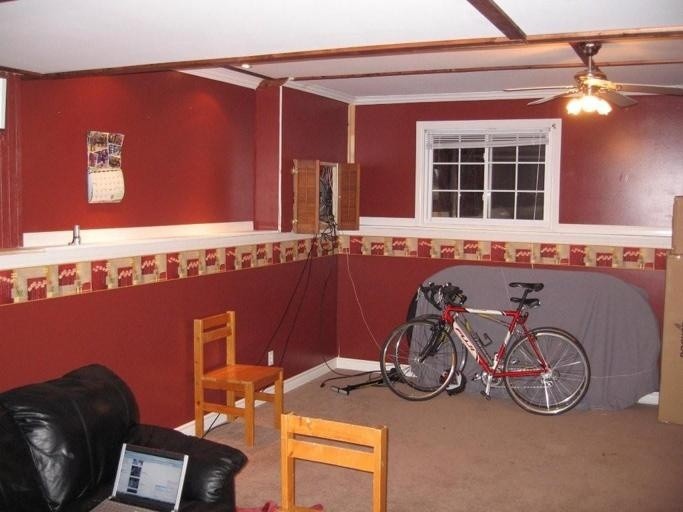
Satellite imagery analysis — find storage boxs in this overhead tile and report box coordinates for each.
[672,196,683,255]
[657,256,683,425]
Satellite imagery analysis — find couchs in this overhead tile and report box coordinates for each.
[0,365,248,512]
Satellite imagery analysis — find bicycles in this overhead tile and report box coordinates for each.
[380,280,591,416]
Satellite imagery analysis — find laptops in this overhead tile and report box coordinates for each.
[88,442,190,512]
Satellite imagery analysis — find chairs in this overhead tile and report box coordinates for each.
[280,411,387,512]
[193,310,284,447]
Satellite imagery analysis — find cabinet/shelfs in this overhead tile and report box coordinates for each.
[292,160,361,236]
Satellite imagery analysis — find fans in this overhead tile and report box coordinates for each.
[504,42,683,107]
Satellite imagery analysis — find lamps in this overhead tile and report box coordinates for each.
[565,94,612,117]
